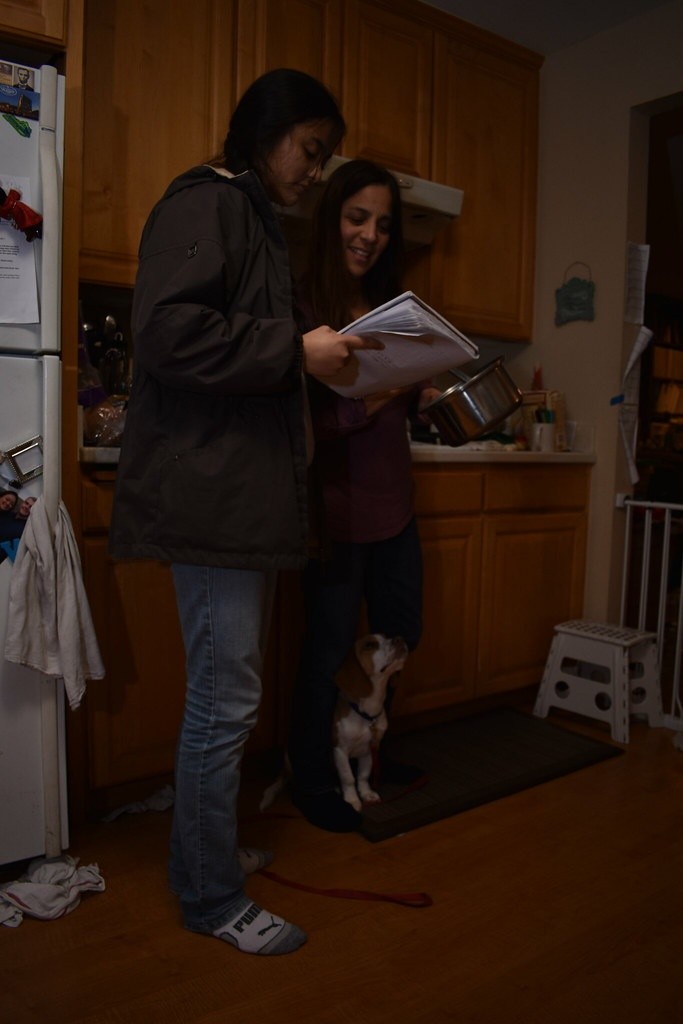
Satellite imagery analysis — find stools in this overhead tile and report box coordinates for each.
[533,619,664,741]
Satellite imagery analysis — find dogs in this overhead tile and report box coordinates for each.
[256,631,408,815]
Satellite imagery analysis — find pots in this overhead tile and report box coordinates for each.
[421,354,523,448]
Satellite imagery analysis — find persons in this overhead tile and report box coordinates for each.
[107,68,448,957]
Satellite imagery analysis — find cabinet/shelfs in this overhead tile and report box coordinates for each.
[78,1,594,820]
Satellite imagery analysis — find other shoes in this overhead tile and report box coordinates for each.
[347,736,431,789]
[280,767,358,830]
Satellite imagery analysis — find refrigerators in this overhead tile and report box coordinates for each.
[0,62,67,861]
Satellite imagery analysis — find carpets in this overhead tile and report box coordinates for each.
[360,706,626,844]
